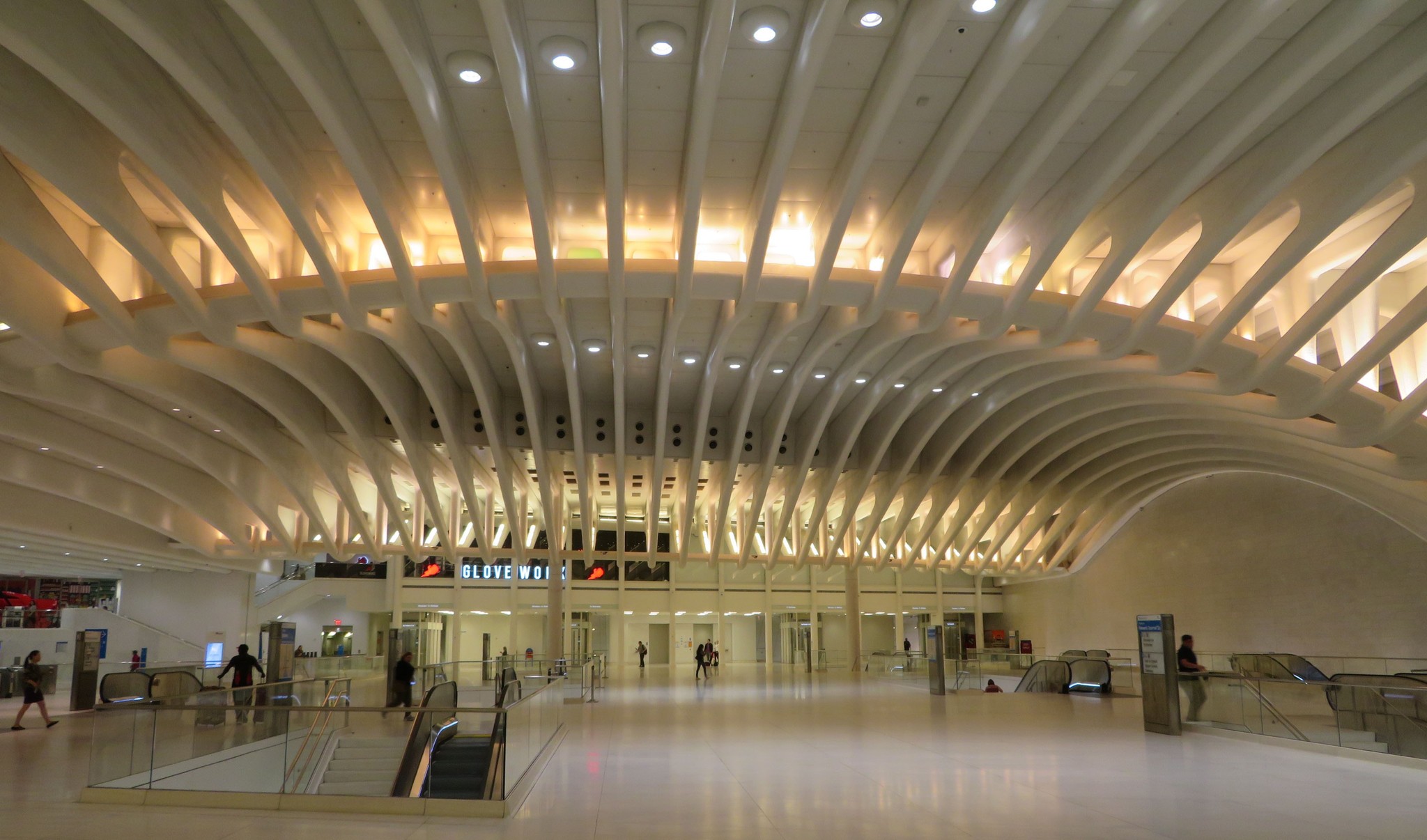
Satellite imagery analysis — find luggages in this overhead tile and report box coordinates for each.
[194,678,227,728]
[252,678,266,723]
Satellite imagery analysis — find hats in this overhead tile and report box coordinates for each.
[236,644,249,650]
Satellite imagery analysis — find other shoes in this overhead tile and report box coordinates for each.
[696,677,701,680]
[381,708,388,719]
[404,715,415,721]
[46,720,59,729]
[11,726,25,731]
[705,676,710,679]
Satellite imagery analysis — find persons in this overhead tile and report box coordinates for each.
[129,650,140,672]
[982,679,1003,693]
[295,645,303,657]
[10,650,60,731]
[27,600,37,627]
[704,639,719,666]
[499,647,507,670]
[1177,635,1208,721]
[217,644,266,724]
[382,651,415,721]
[695,644,711,680]
[636,640,646,667]
[904,637,911,654]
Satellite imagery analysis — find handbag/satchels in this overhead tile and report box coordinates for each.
[695,656,698,660]
[23,667,44,682]
[392,683,407,693]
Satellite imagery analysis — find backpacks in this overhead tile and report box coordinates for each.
[642,645,647,655]
